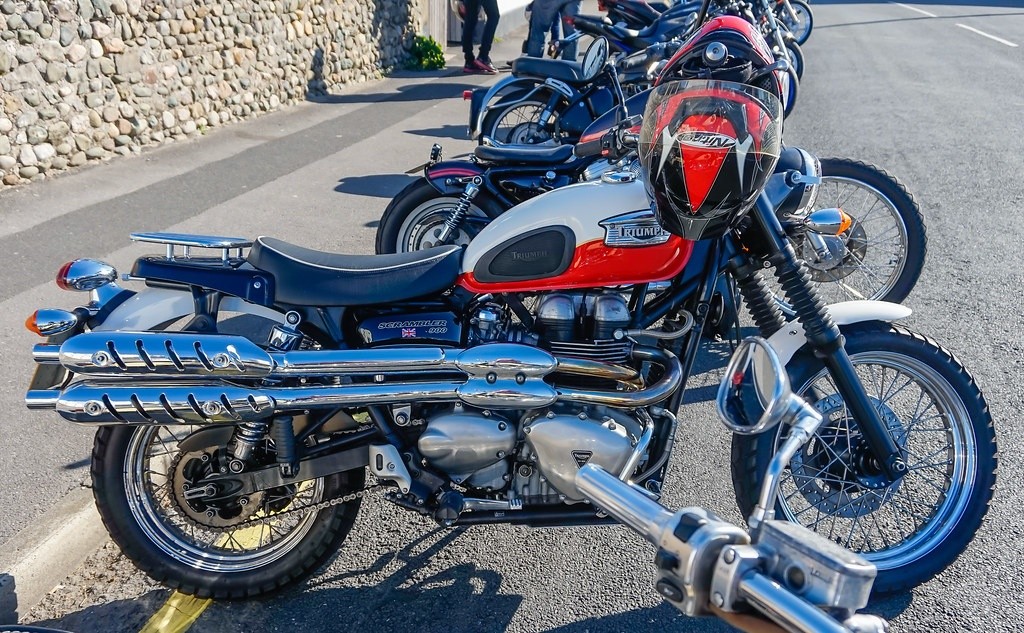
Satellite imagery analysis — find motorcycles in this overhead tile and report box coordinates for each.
[23,0,1000,633]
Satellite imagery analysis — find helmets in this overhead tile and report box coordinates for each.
[449,0,467,23]
[638,14,783,242]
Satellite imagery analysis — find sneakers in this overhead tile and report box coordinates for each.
[464,60,496,74]
[477,56,498,73]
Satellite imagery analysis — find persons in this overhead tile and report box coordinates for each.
[460,0,500,74]
[507,0,581,66]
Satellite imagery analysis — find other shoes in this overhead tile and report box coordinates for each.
[507,60,514,67]
